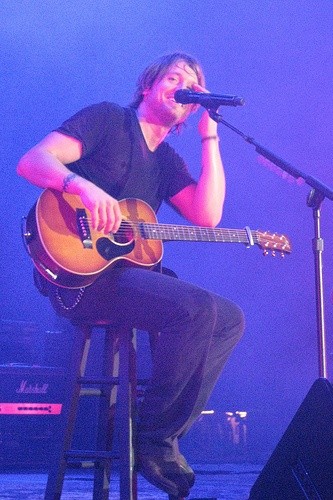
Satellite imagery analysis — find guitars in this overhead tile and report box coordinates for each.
[26,187,292,290]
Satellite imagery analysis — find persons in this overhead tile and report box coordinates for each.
[17,52,246,497]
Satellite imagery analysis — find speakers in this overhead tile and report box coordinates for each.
[249,375,333,499]
[0,364,68,465]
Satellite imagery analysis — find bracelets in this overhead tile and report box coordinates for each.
[199,135,219,142]
[59,171,78,192]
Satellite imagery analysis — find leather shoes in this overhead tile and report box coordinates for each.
[156,451,194,489]
[134,455,190,498]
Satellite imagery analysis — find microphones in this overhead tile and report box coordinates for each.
[174,88,246,108]
